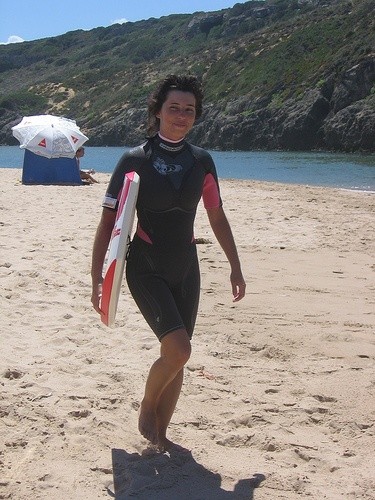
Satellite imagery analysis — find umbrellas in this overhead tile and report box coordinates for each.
[10,115,89,159]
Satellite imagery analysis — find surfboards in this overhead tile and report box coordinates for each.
[101,171,139,327]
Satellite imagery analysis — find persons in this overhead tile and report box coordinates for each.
[90,73,247,455]
[75,147,98,184]
